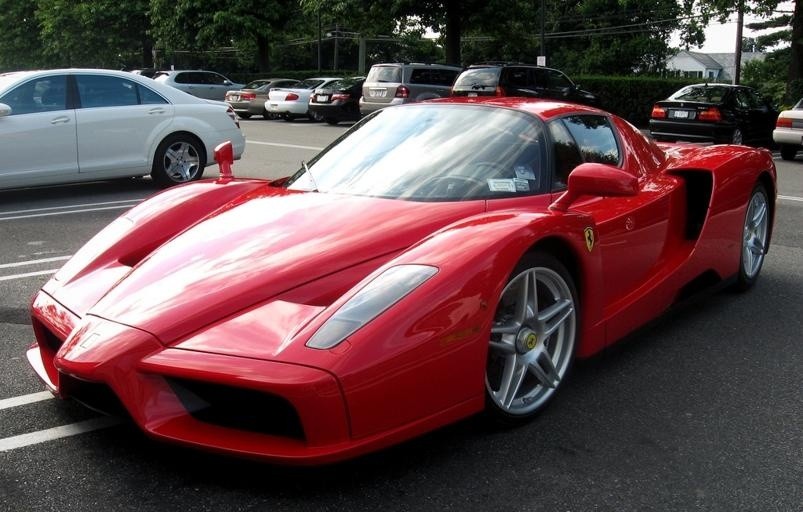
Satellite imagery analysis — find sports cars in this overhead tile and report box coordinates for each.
[25,96,781,467]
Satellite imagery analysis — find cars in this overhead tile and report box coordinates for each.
[0,69,246,189]
